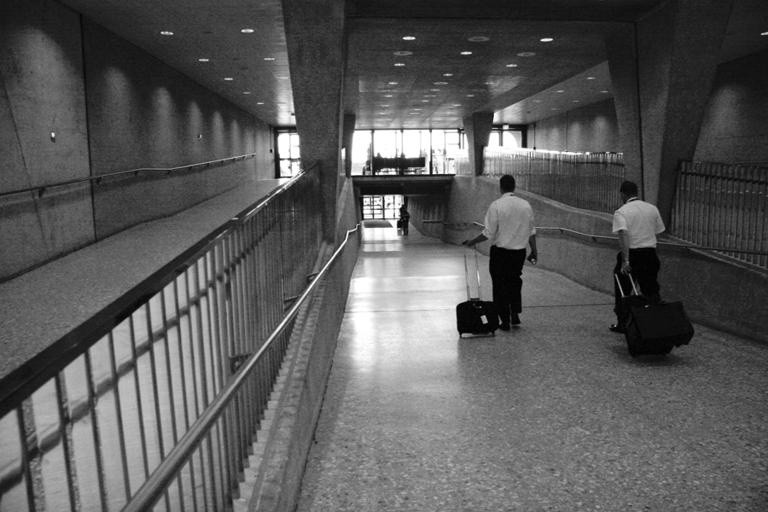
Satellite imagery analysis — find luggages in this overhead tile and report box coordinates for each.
[614,269,693,356]
[457,241,499,337]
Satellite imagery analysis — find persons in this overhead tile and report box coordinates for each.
[460,174,538,331]
[398,204,405,212]
[605,180,666,335]
[400,209,410,236]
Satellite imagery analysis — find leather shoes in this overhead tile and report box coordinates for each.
[499,314,521,330]
[608,325,625,333]
